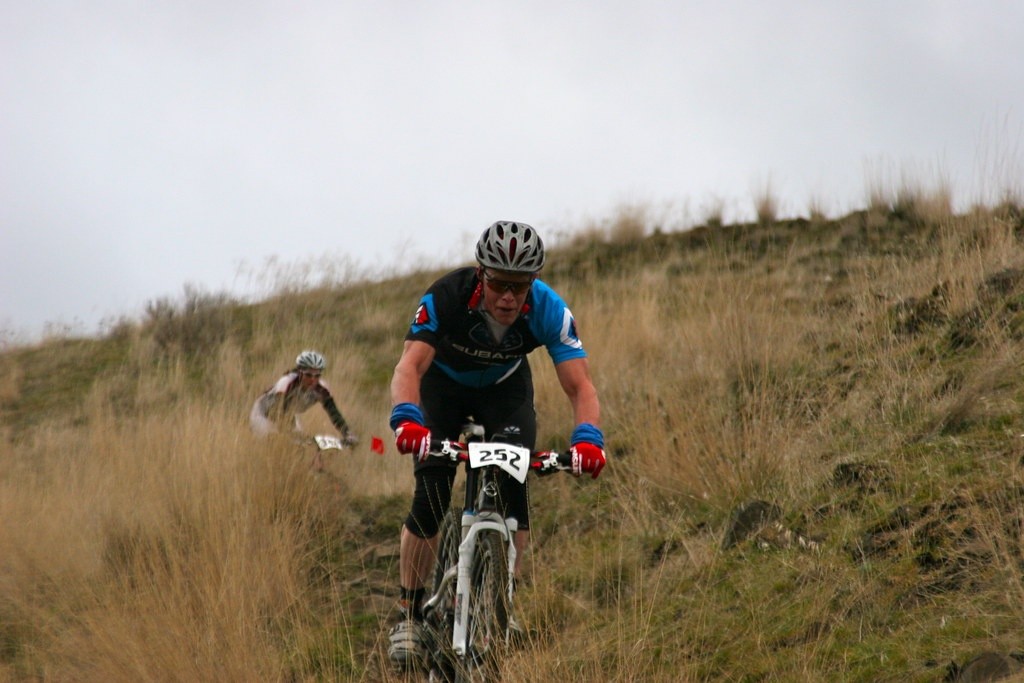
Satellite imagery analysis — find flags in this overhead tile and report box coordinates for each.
[370,436,384,454]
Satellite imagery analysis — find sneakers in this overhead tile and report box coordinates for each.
[509,617,524,636]
[388,620,423,665]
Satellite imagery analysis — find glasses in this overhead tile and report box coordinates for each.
[483,270,534,294]
[298,368,323,379]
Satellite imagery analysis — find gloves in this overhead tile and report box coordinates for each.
[344,431,358,445]
[564,441,605,479]
[393,419,432,461]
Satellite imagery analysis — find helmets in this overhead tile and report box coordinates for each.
[476,221,547,283]
[296,350,326,369]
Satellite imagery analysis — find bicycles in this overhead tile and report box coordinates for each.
[408,417,573,683]
[288,432,353,505]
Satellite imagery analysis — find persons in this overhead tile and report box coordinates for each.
[250,351,358,448]
[388,221,607,658]
